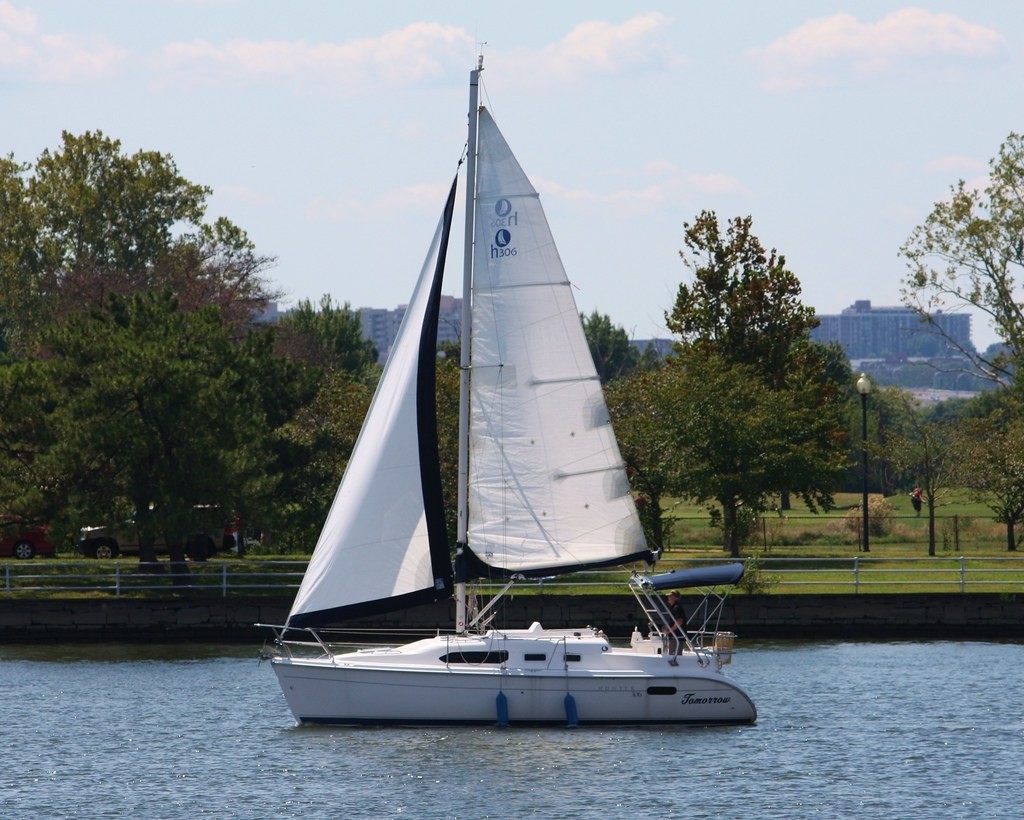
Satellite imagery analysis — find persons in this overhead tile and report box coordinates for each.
[908,487,927,518]
[663,591,687,656]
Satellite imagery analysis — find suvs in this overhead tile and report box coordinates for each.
[76,501,237,562]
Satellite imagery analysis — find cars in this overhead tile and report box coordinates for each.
[0,512,56,561]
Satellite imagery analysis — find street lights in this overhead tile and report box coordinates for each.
[856,373,874,553]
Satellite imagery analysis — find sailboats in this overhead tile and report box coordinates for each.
[252,39,760,724]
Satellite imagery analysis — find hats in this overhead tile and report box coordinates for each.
[665,590,680,597]
